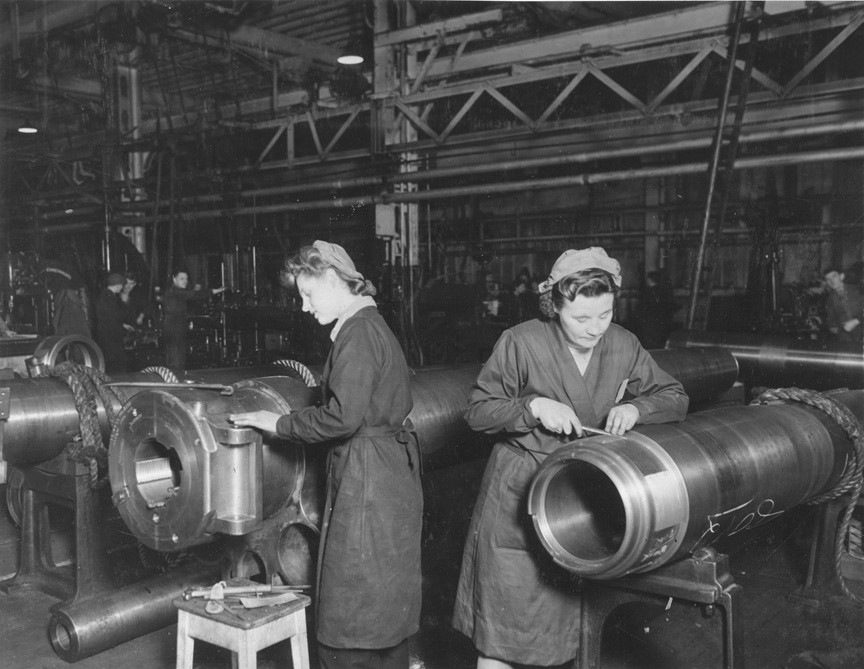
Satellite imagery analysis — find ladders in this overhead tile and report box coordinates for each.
[687,1,766,333]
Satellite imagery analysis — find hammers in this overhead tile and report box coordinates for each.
[182,584,271,601]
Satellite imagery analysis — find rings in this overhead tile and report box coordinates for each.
[556,428,562,432]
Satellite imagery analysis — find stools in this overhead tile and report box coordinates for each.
[173,575,313,669]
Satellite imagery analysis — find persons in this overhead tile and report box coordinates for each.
[636,270,666,321]
[226,239,425,669]
[95,272,137,375]
[450,244,691,669]
[506,269,545,326]
[158,269,227,370]
[823,265,864,344]
[116,272,146,335]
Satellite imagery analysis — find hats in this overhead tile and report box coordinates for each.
[539,247,624,294]
[104,273,126,285]
